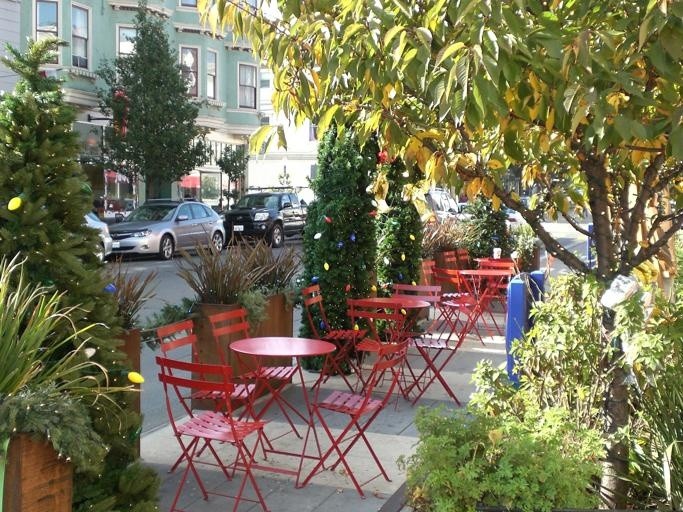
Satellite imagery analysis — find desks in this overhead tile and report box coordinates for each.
[227,337,338,488]
[359,297,433,403]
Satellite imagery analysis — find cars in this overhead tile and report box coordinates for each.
[424,188,530,224]
[81,192,309,262]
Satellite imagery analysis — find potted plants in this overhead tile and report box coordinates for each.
[0,250,143,512]
[515,223,541,272]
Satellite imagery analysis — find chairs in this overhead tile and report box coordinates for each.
[297,352,407,500]
[301,284,370,395]
[154,356,268,512]
[378,284,443,392]
[404,291,485,410]
[210,307,303,451]
[156,318,269,464]
[345,296,412,403]
[421,249,523,348]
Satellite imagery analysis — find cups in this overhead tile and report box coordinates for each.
[493,248,501,259]
